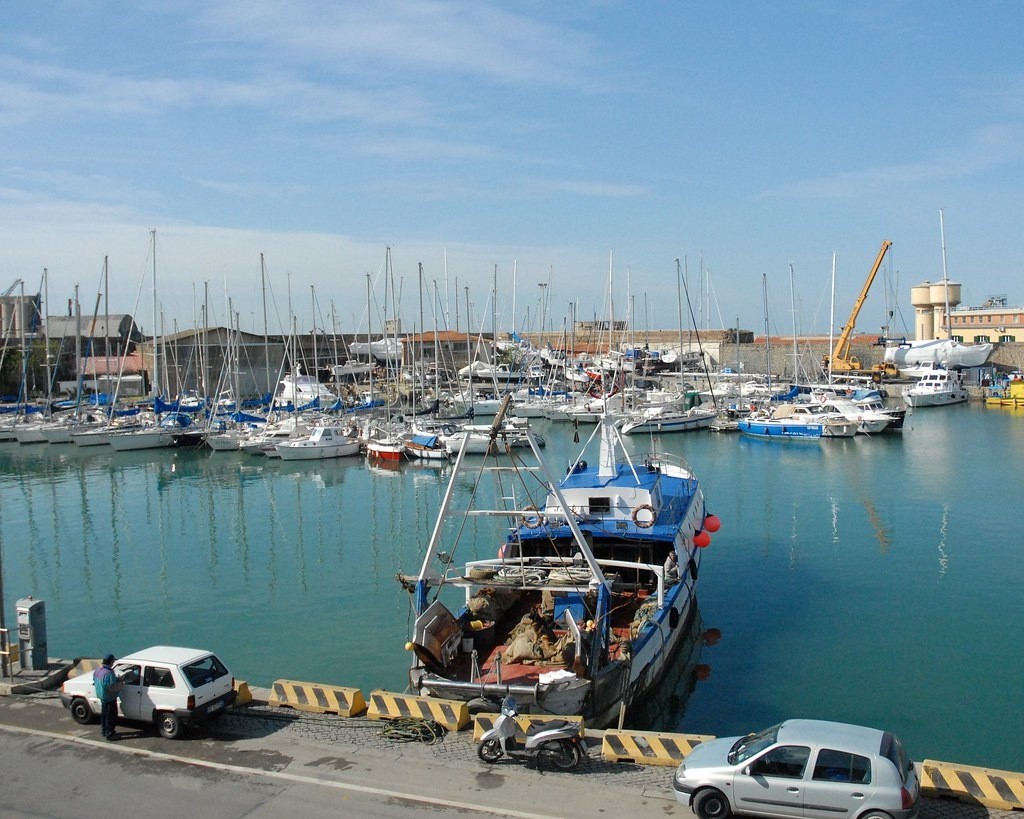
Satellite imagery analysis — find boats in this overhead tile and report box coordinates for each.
[397,358,706,729]
[898,359,969,407]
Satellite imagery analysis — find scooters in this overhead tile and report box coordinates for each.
[478,695,589,771]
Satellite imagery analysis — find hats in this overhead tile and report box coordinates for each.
[104,654,118,661]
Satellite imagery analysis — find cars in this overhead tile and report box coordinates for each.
[59,645,237,739]
[672,720,921,819]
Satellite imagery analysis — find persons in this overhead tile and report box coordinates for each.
[93,654,124,740]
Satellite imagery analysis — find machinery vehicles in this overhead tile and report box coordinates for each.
[819,240,900,384]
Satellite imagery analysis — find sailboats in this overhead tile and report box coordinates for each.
[0,226,907,464]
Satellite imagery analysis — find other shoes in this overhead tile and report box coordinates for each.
[106,734,121,741]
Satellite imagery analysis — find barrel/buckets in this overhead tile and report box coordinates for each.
[462,637,473,652]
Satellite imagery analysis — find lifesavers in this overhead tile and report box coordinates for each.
[521,505,543,529]
[632,504,657,528]
[588,368,619,400]
[690,559,697,580]
[820,395,826,403]
[824,427,828,433]
[670,607,679,628]
